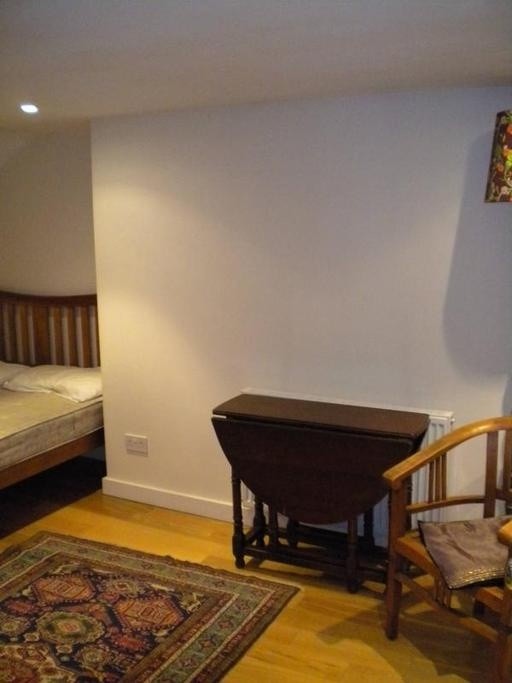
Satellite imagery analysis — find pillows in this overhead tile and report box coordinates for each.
[1,359,32,385]
[3,364,103,402]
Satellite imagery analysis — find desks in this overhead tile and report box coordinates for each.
[211,393,430,593]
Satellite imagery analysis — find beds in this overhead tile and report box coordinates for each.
[0,289,105,489]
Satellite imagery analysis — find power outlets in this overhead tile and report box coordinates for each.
[125,433,149,453]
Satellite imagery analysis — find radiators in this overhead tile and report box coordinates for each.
[240,388,454,548]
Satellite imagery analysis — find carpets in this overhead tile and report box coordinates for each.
[1,530,301,682]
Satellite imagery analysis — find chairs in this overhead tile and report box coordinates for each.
[382,415,511,682]
